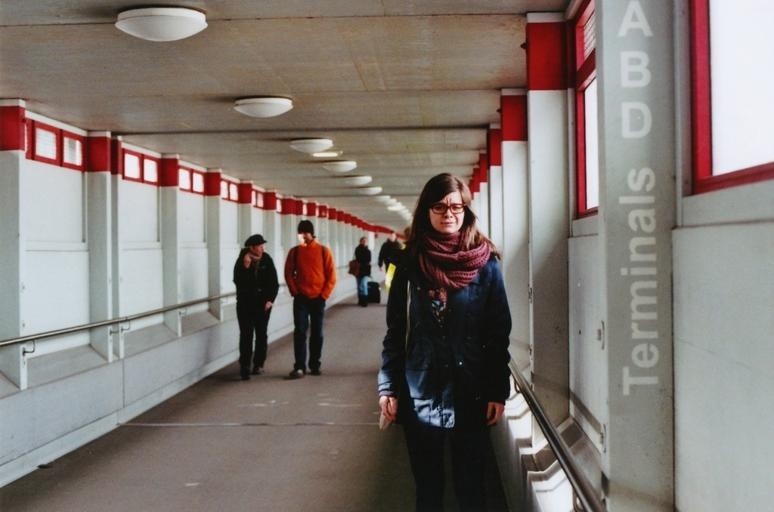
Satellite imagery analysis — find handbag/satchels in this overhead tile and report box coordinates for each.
[349,261,361,277]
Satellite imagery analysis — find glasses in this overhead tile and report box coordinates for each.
[431,202,465,214]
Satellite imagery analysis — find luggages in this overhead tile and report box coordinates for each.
[368,282,380,302]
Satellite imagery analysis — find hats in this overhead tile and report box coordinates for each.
[245,234,267,247]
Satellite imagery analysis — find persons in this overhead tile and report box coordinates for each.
[233,221,412,379]
[377,172,512,512]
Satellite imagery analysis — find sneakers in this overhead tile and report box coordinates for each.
[310,366,320,375]
[290,367,305,375]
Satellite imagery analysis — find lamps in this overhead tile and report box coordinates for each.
[234,97,294,118]
[114,6,208,43]
[289,138,333,153]
[344,176,414,223]
[323,161,357,173]
